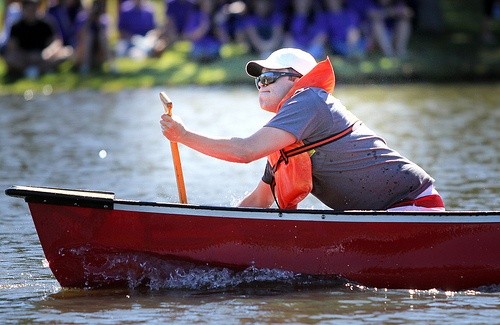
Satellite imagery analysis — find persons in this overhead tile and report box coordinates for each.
[161,47,446,212]
[0,0,412,82]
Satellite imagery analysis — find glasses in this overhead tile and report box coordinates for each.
[255,71,301,90]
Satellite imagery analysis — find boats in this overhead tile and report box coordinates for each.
[5,183,496,288]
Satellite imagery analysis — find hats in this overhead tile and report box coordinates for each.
[246,48,318,79]
